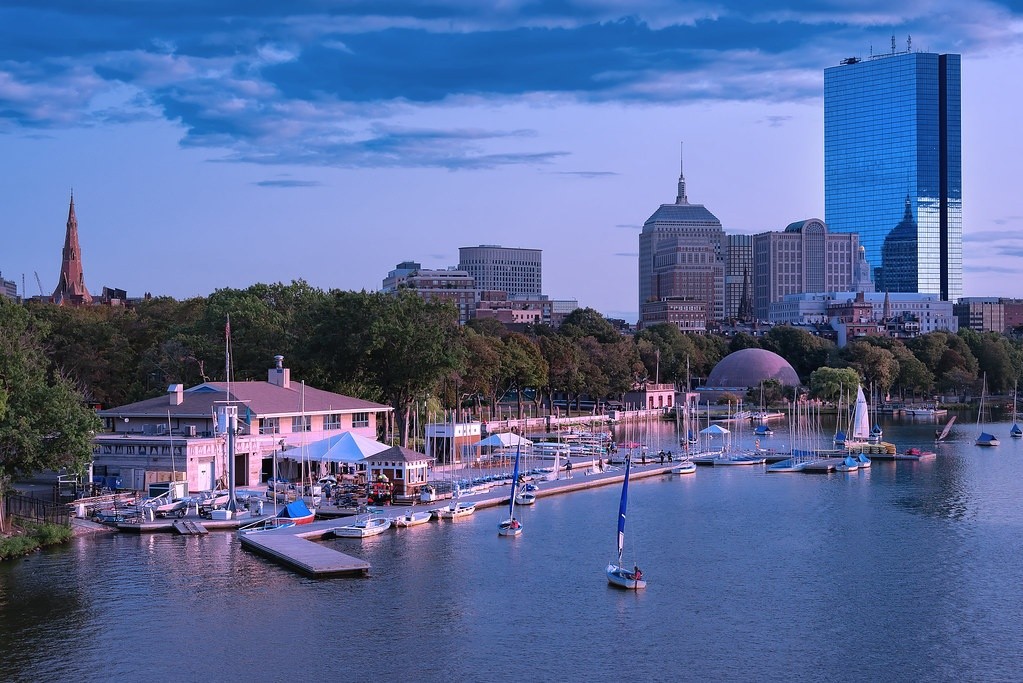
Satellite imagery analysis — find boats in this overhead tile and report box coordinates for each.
[334,507,391,538]
[390,511,432,527]
[266,478,321,505]
[904,408,949,415]
[430,502,478,519]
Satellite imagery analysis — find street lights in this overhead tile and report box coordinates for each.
[147,373,156,392]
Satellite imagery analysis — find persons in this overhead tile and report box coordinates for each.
[624,453,630,468]
[659,450,665,465]
[629,566,641,580]
[324,481,332,506]
[668,451,672,463]
[606,442,616,456]
[509,518,518,529]
[599,457,604,473]
[561,459,572,479]
[641,450,646,466]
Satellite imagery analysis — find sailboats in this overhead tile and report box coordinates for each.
[604,456,647,589]
[974,370,1000,446]
[497,442,523,536]
[272,378,317,532]
[428,381,883,503]
[236,427,296,540]
[1011,378,1022,436]
[933,415,957,444]
[670,422,696,473]
[199,406,229,508]
[515,427,535,505]
[143,410,191,513]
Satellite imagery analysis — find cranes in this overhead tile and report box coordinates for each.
[33,271,43,296]
[22,274,25,298]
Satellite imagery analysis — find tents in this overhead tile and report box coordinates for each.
[699,424,730,445]
[277,431,393,480]
[472,433,533,460]
